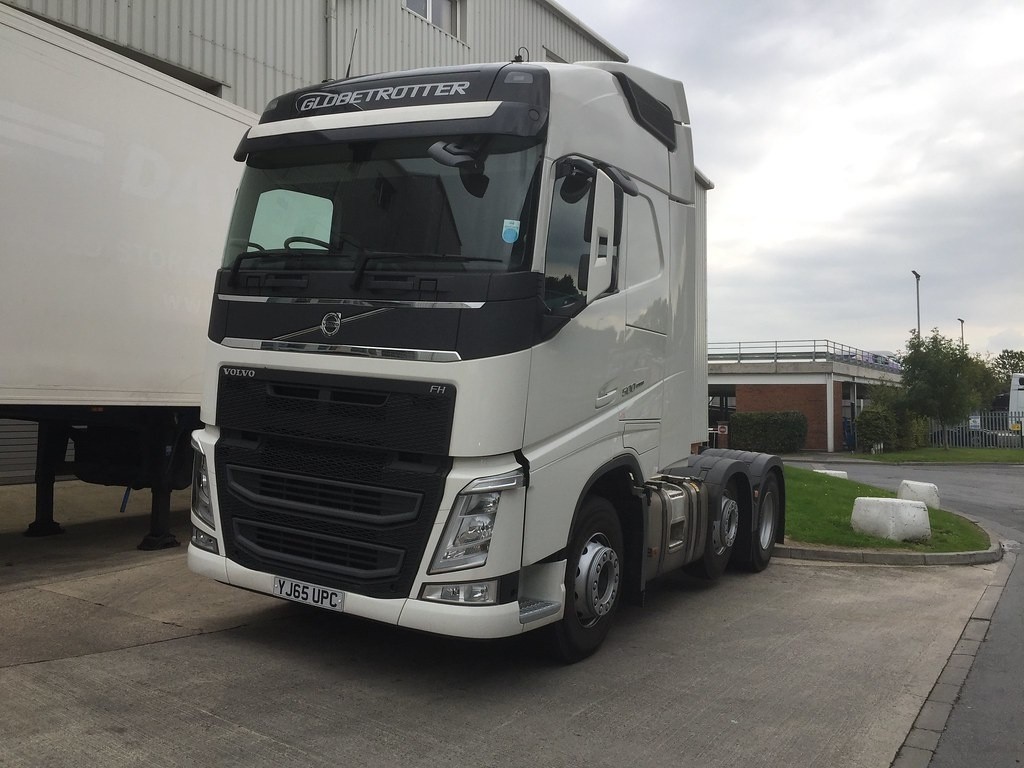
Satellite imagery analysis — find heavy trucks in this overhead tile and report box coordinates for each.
[186,31,789,662]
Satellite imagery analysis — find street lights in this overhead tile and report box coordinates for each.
[957,318,965,349]
[912,270,921,342]
